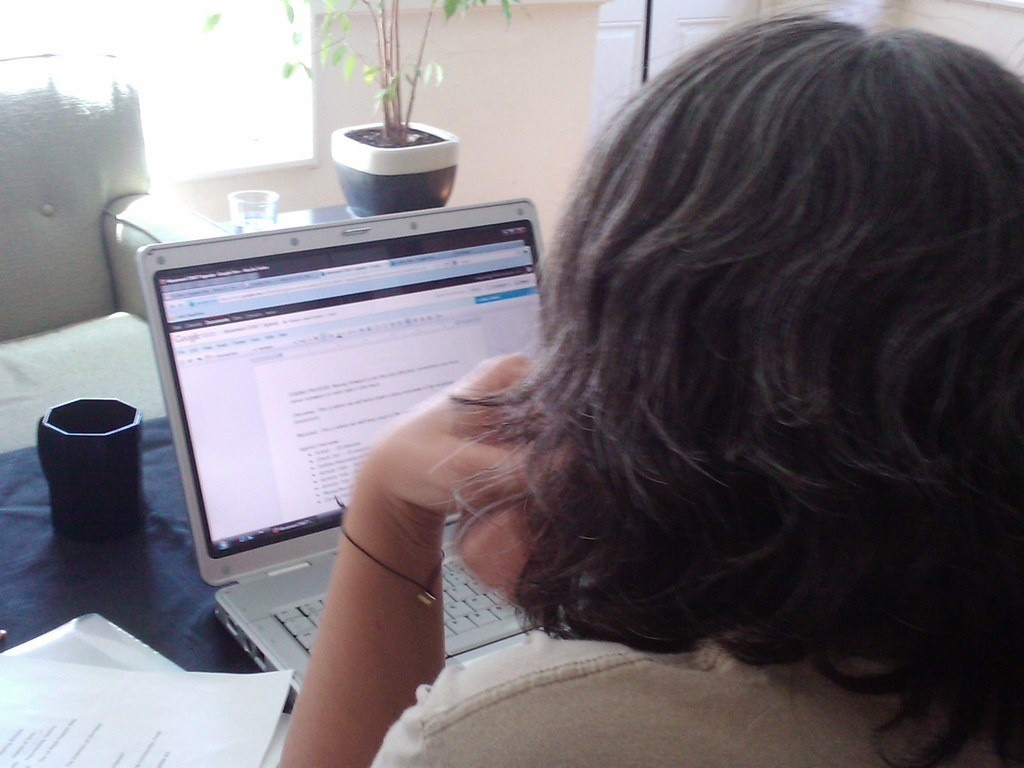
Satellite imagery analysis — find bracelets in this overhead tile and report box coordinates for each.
[332,497,447,608]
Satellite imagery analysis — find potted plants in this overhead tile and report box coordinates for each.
[203,0,534,217]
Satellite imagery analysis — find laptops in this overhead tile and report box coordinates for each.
[136,199,549,710]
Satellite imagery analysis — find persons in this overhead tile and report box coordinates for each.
[277,12,1024,768]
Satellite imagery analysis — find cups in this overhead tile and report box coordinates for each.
[228,190,281,235]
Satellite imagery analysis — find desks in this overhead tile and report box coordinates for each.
[97,155,353,327]
[1,409,292,672]
